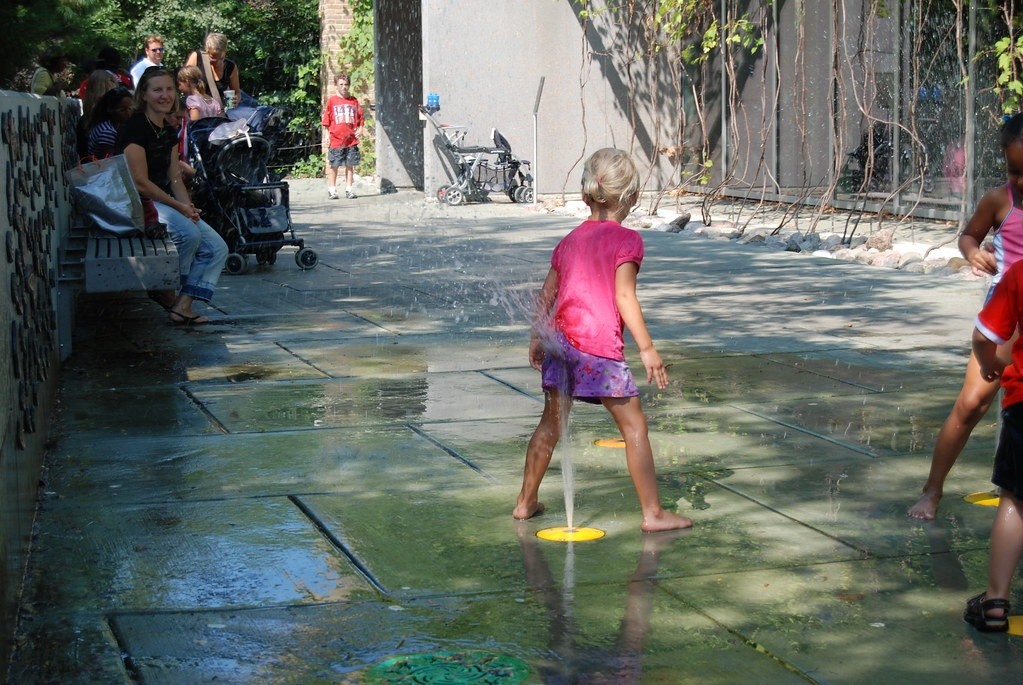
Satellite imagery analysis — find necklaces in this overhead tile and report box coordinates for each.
[145,113,162,138]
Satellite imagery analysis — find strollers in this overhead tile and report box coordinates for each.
[415,92,535,206]
[186,114,321,279]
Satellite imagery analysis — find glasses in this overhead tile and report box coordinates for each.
[143,66,166,75]
[147,48,164,52]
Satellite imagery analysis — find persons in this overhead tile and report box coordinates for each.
[31,46,65,97]
[516,520,694,685]
[513,149,694,530]
[77,32,241,165]
[322,72,364,199]
[114,70,228,326]
[908,111,1023,519]
[963,260,1023,633]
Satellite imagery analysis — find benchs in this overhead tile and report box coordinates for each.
[56,212,179,360]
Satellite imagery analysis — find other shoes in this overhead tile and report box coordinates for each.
[346,191,357,199]
[328,189,338,199]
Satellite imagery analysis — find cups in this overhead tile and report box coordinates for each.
[223,90,236,109]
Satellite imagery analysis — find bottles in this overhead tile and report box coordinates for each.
[428,93,440,106]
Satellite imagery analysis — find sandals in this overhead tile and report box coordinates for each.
[164,310,210,327]
[963,591,1011,632]
[147,289,176,310]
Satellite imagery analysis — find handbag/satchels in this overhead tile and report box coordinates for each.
[64,154,145,237]
